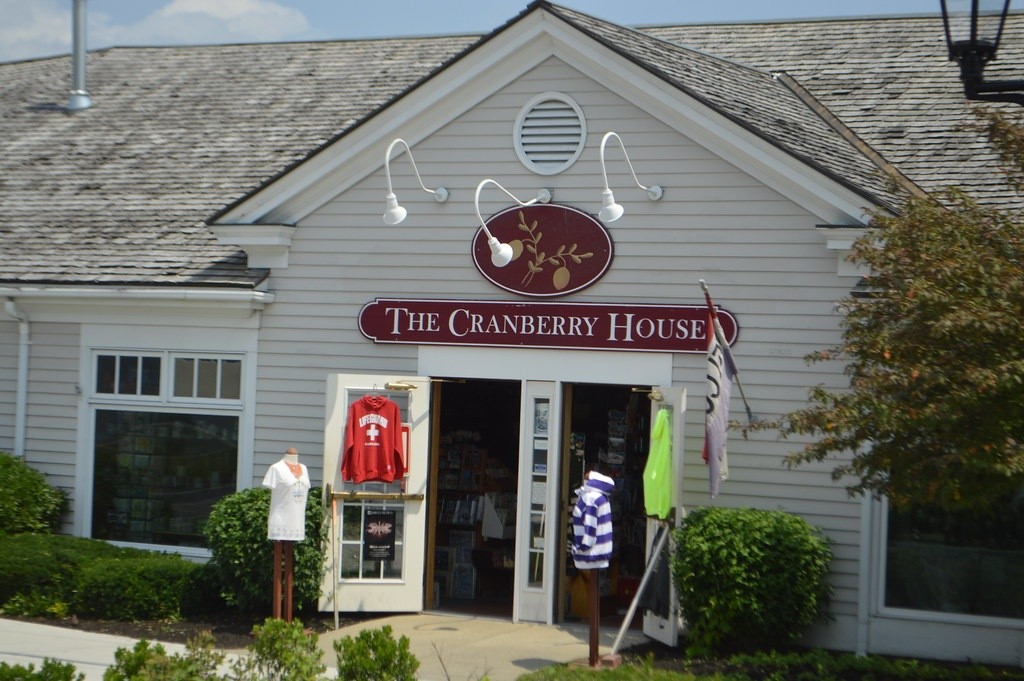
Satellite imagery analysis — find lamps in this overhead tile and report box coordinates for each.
[383,138,449,225]
[598,132,662,224]
[473,179,552,267]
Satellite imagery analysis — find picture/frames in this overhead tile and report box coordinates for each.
[432,427,517,601]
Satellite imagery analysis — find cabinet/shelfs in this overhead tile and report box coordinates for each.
[435,458,515,553]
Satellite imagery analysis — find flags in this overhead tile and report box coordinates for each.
[701,297,737,499]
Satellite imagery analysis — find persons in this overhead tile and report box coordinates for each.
[263,448,310,541]
[573,472,615,568]
